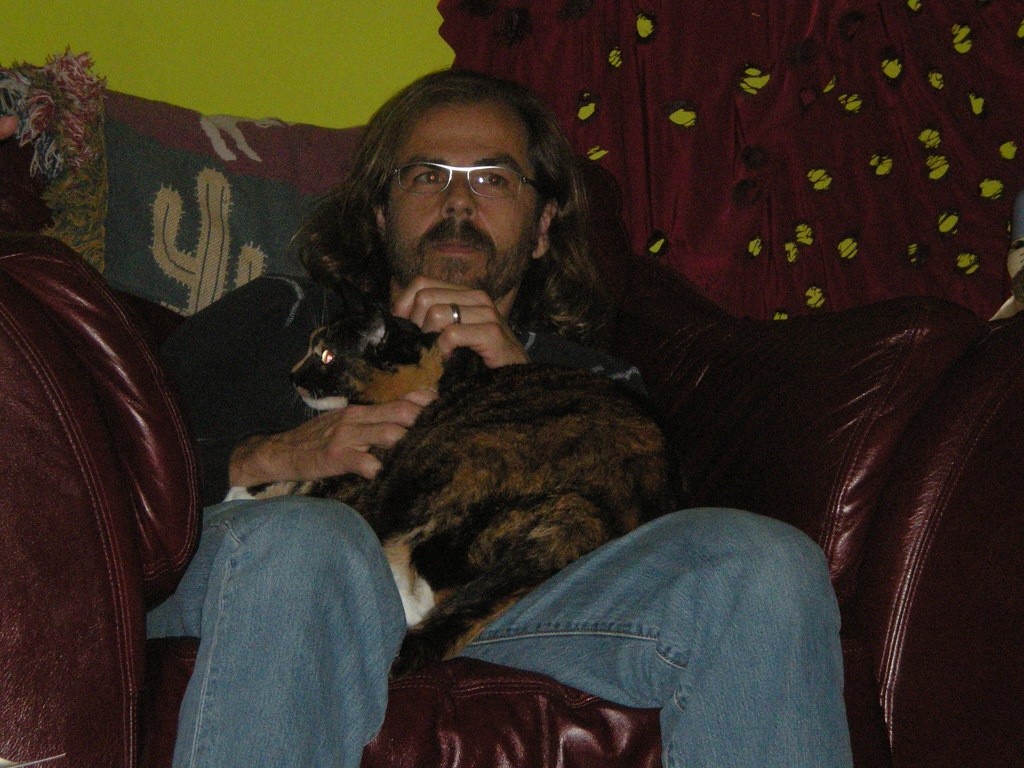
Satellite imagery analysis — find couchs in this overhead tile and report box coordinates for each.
[0,66,1020,764]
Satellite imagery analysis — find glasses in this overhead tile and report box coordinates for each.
[390,161,540,198]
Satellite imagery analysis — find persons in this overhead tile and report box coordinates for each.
[145,68,852,768]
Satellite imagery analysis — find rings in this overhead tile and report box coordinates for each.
[450,303,461,324]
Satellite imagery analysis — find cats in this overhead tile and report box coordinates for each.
[222,296,675,681]
